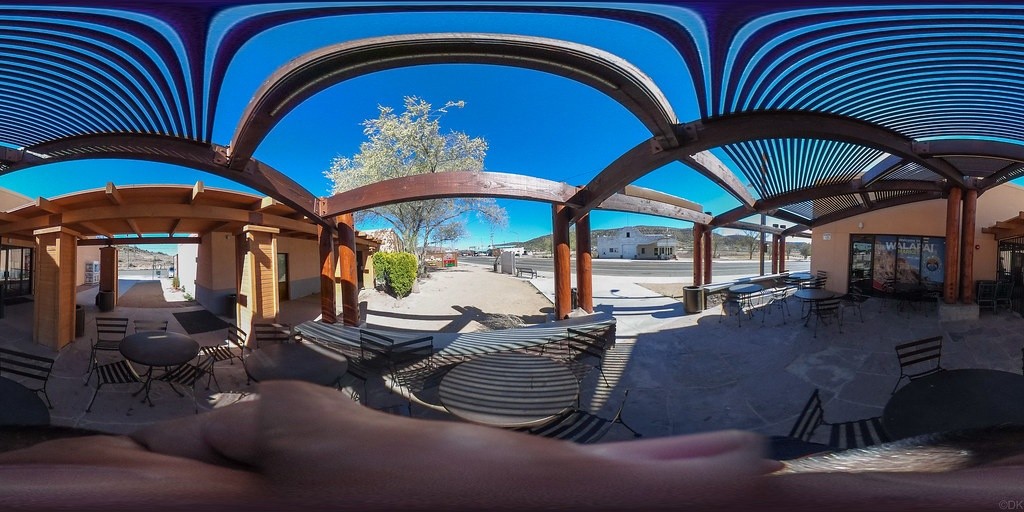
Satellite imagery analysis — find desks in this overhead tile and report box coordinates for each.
[244,342,349,392]
[0,376,51,453]
[879,368,1023,442]
[438,353,581,433]
[118,331,200,408]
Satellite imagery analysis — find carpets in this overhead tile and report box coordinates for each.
[172,310,232,335]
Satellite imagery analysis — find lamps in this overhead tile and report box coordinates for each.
[858,222,864,231]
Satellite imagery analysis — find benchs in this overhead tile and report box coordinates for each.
[516,266,538,279]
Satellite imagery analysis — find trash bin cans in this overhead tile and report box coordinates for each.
[76,303,86,337]
[683,286,705,313]
[97,290,114,312]
[227,294,236,318]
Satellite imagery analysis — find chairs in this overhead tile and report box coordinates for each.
[533,389,643,445]
[254,323,292,350]
[140,344,223,415]
[337,328,395,407]
[717,269,871,338]
[784,388,890,467]
[85,316,130,374]
[974,279,1016,316]
[876,277,948,319]
[83,338,156,414]
[198,321,250,390]
[383,336,447,418]
[891,335,948,395]
[553,328,612,408]
[0,346,55,411]
[133,319,169,334]
